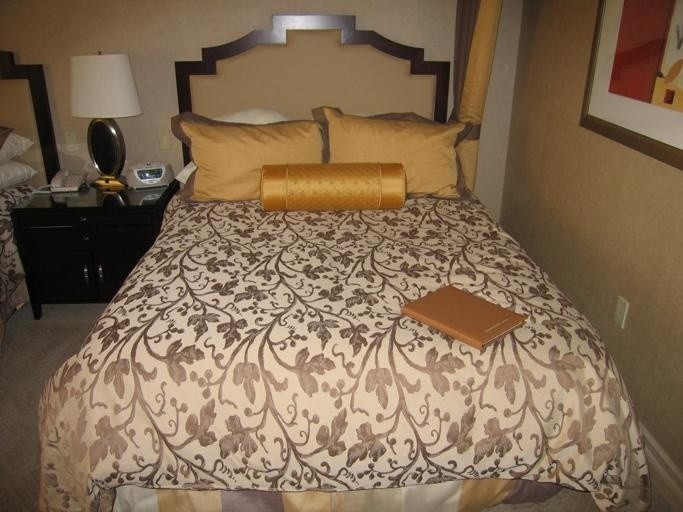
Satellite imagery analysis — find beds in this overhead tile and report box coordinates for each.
[36,15,656,511]
[0,49,61,336]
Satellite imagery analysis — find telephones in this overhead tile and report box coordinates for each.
[50,168,86,193]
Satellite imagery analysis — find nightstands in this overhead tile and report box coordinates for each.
[9,171,180,320]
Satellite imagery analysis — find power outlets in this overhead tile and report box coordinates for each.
[613,295,629,331]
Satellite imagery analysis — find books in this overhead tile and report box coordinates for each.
[401,286,524,345]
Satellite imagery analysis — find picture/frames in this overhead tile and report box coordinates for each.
[578,0,683,171]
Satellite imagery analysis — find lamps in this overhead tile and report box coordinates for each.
[67,54,143,192]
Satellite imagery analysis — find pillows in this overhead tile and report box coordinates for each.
[1,125,34,161]
[311,104,472,200]
[256,162,409,214]
[168,111,330,206]
[0,160,38,188]
